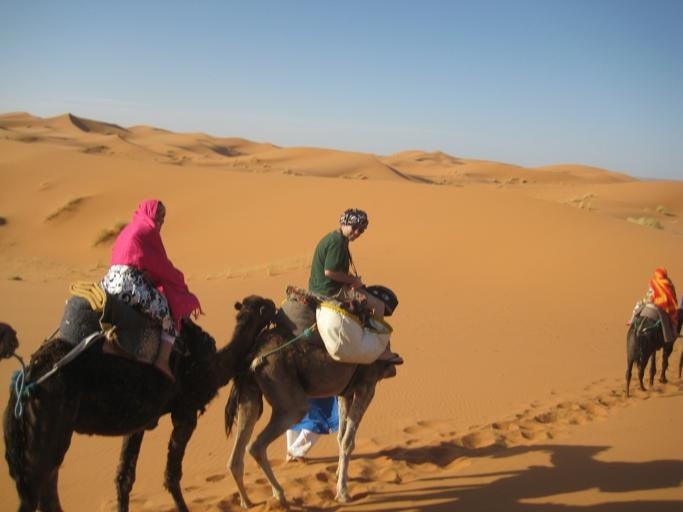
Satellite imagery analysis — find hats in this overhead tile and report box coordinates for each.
[339,208,368,228]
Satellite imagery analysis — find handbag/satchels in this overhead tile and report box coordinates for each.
[315,300,394,364]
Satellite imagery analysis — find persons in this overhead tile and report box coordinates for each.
[627,264,682,339]
[98,198,200,381]
[285,394,340,463]
[306,206,406,366]
